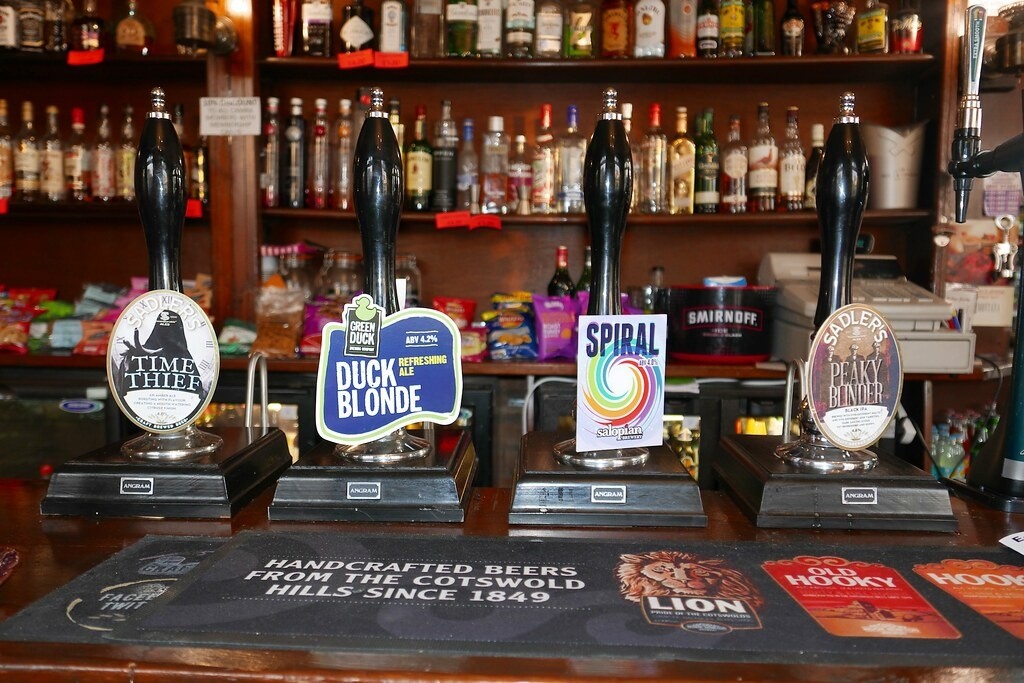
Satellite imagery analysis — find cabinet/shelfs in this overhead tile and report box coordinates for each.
[228,0,985,474]
[0,0,234,369]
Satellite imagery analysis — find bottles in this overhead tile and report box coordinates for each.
[257,91,826,214]
[1,97,207,202]
[931,401,999,479]
[650,265,665,301]
[0,0,152,54]
[577,245,595,292]
[253,0,926,60]
[547,242,576,297]
